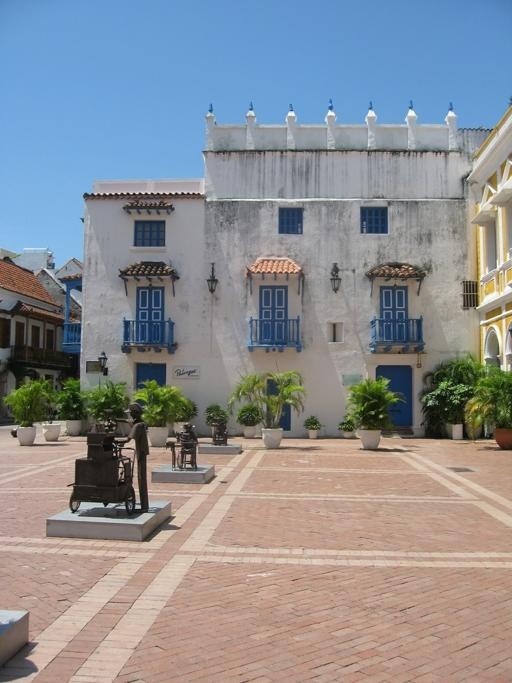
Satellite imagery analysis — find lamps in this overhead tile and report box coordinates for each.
[207,261,218,293]
[329,261,342,293]
[97,350,110,376]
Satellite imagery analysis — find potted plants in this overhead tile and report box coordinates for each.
[303,412,325,439]
[226,355,307,449]
[421,350,510,452]
[5,379,196,453]
[336,413,355,437]
[238,405,260,437]
[204,403,229,438]
[345,373,408,449]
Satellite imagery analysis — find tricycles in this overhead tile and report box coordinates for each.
[65,428,137,514]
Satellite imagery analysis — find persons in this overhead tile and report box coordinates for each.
[113,403,149,513]
